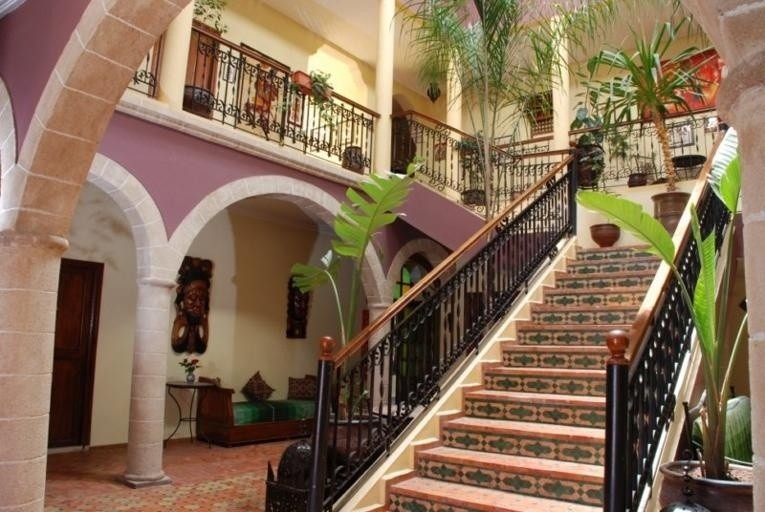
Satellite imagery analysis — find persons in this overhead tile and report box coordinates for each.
[185,279,206,317]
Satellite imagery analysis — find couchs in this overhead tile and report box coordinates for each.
[195,374,321,448]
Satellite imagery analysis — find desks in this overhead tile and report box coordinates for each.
[163,380,215,449]
[672,155,707,181]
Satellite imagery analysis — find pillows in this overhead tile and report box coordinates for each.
[240,370,316,402]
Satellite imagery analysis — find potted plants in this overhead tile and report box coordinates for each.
[290,157,427,481]
[575,157,752,512]
[568,1,705,248]
[452,129,500,206]
[289,67,334,109]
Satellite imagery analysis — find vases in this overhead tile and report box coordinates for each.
[342,147,365,176]
[186,373,194,382]
[183,85,214,119]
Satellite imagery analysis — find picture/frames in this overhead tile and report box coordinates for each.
[667,123,695,149]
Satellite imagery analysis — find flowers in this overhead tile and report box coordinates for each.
[179,358,201,373]
